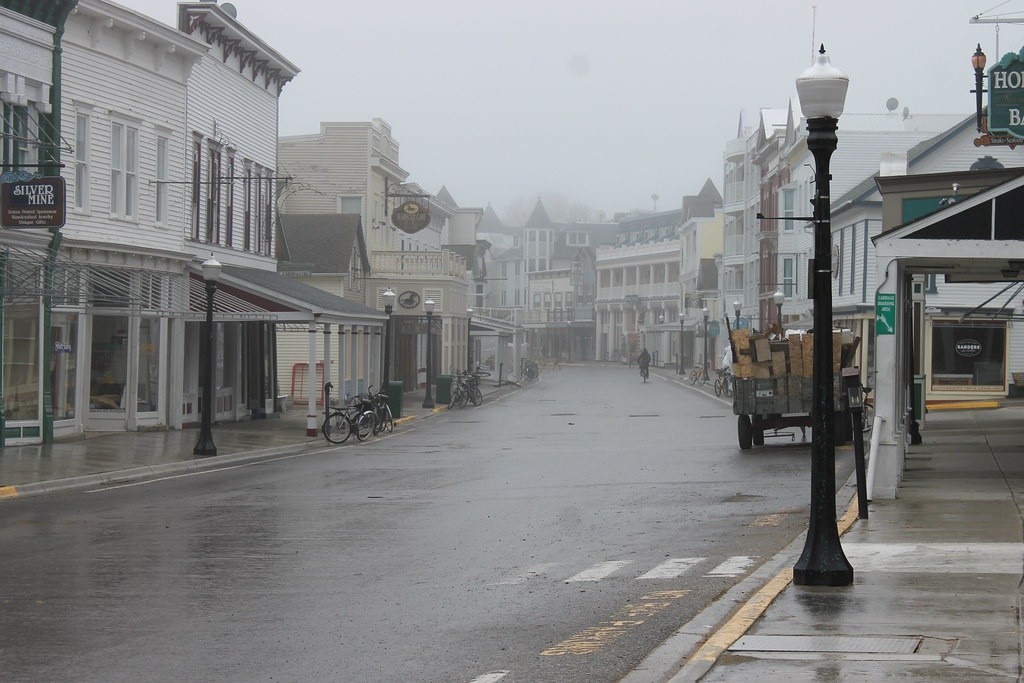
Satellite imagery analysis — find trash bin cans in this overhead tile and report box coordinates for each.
[914,374,927,431]
[436,375,451,404]
[389,381,404,418]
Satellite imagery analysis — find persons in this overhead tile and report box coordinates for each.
[468,354,475,375]
[637,348,650,379]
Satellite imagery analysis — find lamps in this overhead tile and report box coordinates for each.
[938,182,961,207]
[970,43,986,133]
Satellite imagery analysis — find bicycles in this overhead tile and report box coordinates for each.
[713,366,735,398]
[321,384,394,445]
[637,362,650,382]
[447,366,483,410]
[689,363,706,386]
[524,360,540,381]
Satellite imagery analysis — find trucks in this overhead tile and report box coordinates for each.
[723,312,862,449]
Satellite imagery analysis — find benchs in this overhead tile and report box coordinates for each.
[1009,372,1024,398]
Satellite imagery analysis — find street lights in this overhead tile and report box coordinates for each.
[465,305,473,373]
[746,40,853,588]
[733,298,743,330]
[421,297,436,409]
[701,307,710,381]
[772,288,785,335]
[382,286,397,422]
[191,251,222,457]
[677,312,687,375]
